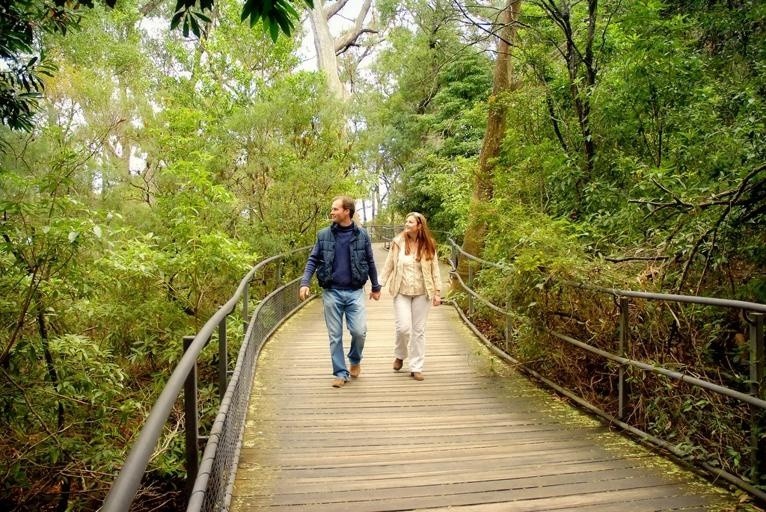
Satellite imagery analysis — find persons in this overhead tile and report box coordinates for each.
[298,193,381,389]
[368,210,442,382]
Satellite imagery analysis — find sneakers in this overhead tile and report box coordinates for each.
[411,372,424,380]
[332,377,345,387]
[394,358,403,370]
[350,363,360,377]
